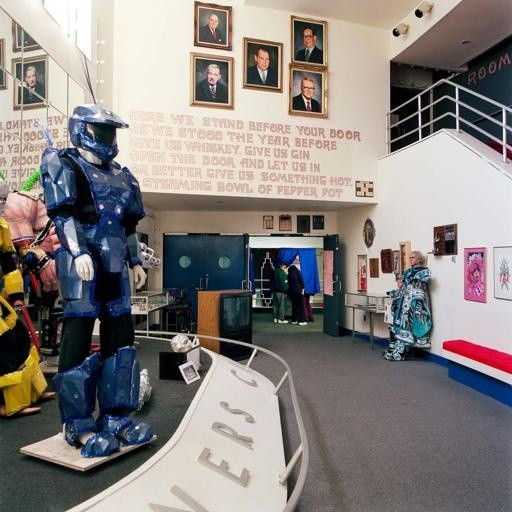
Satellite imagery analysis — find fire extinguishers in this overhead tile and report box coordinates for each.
[361,265,367,290]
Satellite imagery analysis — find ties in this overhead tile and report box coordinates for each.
[261,71,266,85]
[305,101,309,111]
[211,83,215,97]
[305,49,310,62]
[211,29,216,41]
[30,87,33,96]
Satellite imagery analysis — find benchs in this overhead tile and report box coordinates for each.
[441,339,512,407]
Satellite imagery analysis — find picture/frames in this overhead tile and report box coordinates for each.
[190,51,236,110]
[12,54,49,111]
[291,14,328,68]
[464,247,488,303]
[178,361,201,385]
[381,241,411,292]
[194,1,233,51]
[243,36,283,93]
[288,63,329,118]
[12,20,43,53]
[356,180,374,197]
[0,38,8,89]
[434,224,458,256]
[493,246,512,301]
[357,255,368,292]
[370,258,379,278]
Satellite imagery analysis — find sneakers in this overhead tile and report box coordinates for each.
[277,319,289,324]
[291,322,298,325]
[274,319,278,323]
[299,322,307,325]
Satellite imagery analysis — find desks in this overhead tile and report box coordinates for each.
[344,291,390,351]
[131,291,169,338]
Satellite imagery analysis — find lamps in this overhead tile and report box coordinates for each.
[392,23,409,37]
[415,2,433,18]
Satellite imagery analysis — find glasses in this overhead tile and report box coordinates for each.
[304,86,316,91]
[304,35,314,40]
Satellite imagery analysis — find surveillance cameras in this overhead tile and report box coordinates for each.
[393,22,407,37]
[414,0,433,18]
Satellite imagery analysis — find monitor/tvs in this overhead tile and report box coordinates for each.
[198,290,252,361]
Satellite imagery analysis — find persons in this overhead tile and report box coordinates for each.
[1,220,55,416]
[381,251,432,361]
[195,6,322,113]
[16,61,44,104]
[0,104,153,460]
[5,170,62,356]
[41,104,151,457]
[269,263,314,325]
